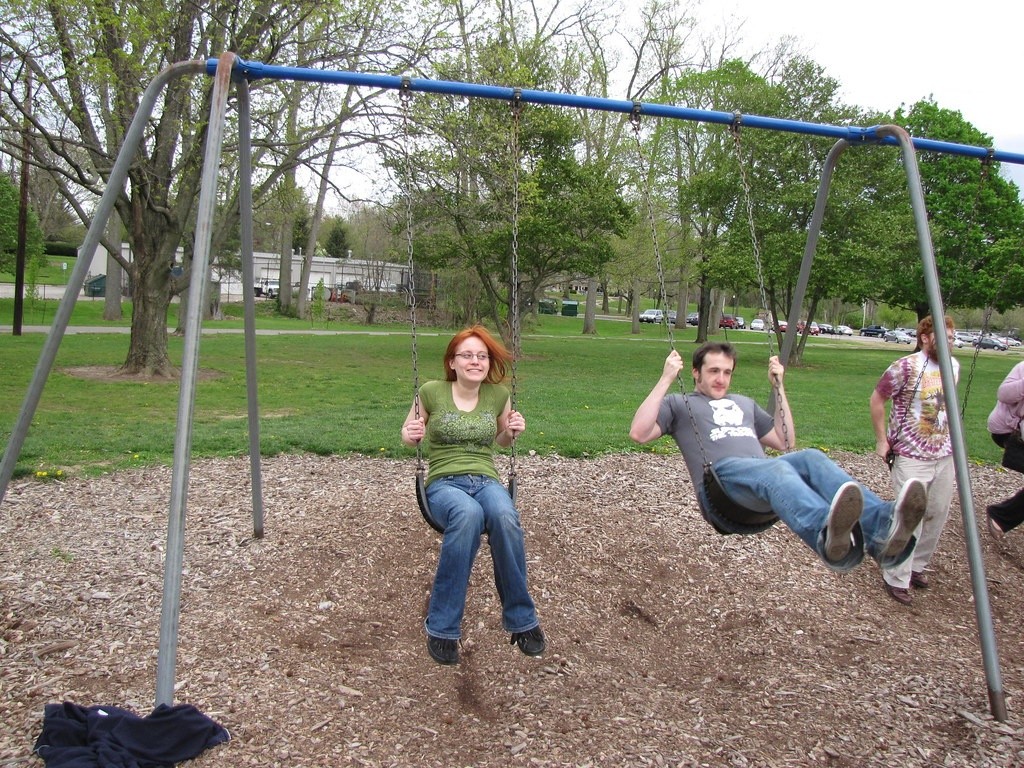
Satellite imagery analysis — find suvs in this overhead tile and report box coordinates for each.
[719,314,739,330]
[639,309,664,325]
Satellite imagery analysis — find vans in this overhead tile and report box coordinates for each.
[799,320,819,336]
[907,329,916,337]
[956,332,977,342]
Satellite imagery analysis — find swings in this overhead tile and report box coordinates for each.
[633,114,790,537]
[885,159,1024,473]
[397,89,526,534]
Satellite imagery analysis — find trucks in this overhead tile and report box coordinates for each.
[254,278,280,299]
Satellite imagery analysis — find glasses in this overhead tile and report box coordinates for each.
[455,351,491,360]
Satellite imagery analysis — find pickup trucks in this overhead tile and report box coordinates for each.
[859,325,891,338]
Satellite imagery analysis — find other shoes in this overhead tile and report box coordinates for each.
[912,569,930,589]
[510,626,546,657]
[824,481,861,566]
[884,478,927,559]
[986,504,1003,542]
[427,631,460,666]
[885,581,913,604]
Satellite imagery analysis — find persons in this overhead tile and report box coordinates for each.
[402,327,545,664]
[629,340,926,569]
[871,316,960,605]
[986,361,1024,541]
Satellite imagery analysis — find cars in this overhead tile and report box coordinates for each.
[735,316,747,329]
[662,310,678,324]
[818,324,835,335]
[972,337,1009,351]
[1001,337,1021,347]
[691,315,699,327]
[895,328,912,337]
[835,325,853,336]
[770,320,788,332]
[686,313,699,323]
[750,319,765,332]
[883,331,912,344]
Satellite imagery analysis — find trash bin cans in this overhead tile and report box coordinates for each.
[562,300,579,317]
[83,274,108,297]
[538,298,559,315]
